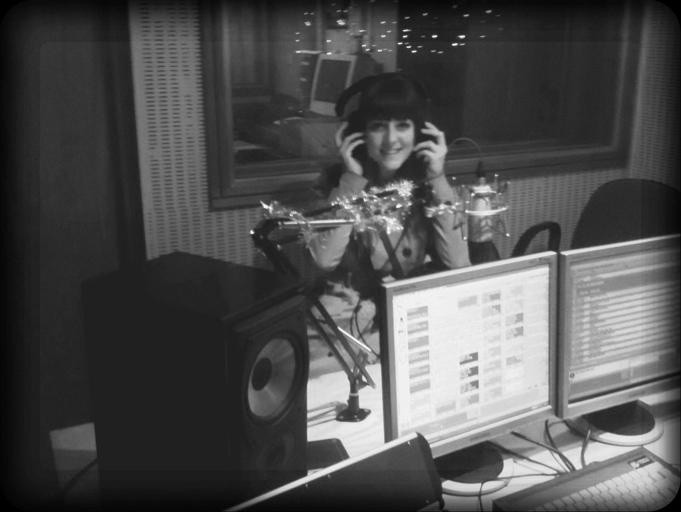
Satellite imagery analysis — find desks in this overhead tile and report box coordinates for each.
[46,363,679,511]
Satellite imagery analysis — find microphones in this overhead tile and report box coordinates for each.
[463,175,500,267]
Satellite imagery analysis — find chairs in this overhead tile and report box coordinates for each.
[512,178,679,263]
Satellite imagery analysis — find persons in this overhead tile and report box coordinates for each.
[306,72,470,382]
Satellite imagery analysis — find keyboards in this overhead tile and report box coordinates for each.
[491,445,681,512]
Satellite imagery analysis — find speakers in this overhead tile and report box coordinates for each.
[81,249,314,512]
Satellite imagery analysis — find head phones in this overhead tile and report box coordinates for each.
[334,71,434,161]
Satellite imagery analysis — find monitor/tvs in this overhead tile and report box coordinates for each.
[378,250,559,496]
[556,232,681,446]
[308,52,380,118]
